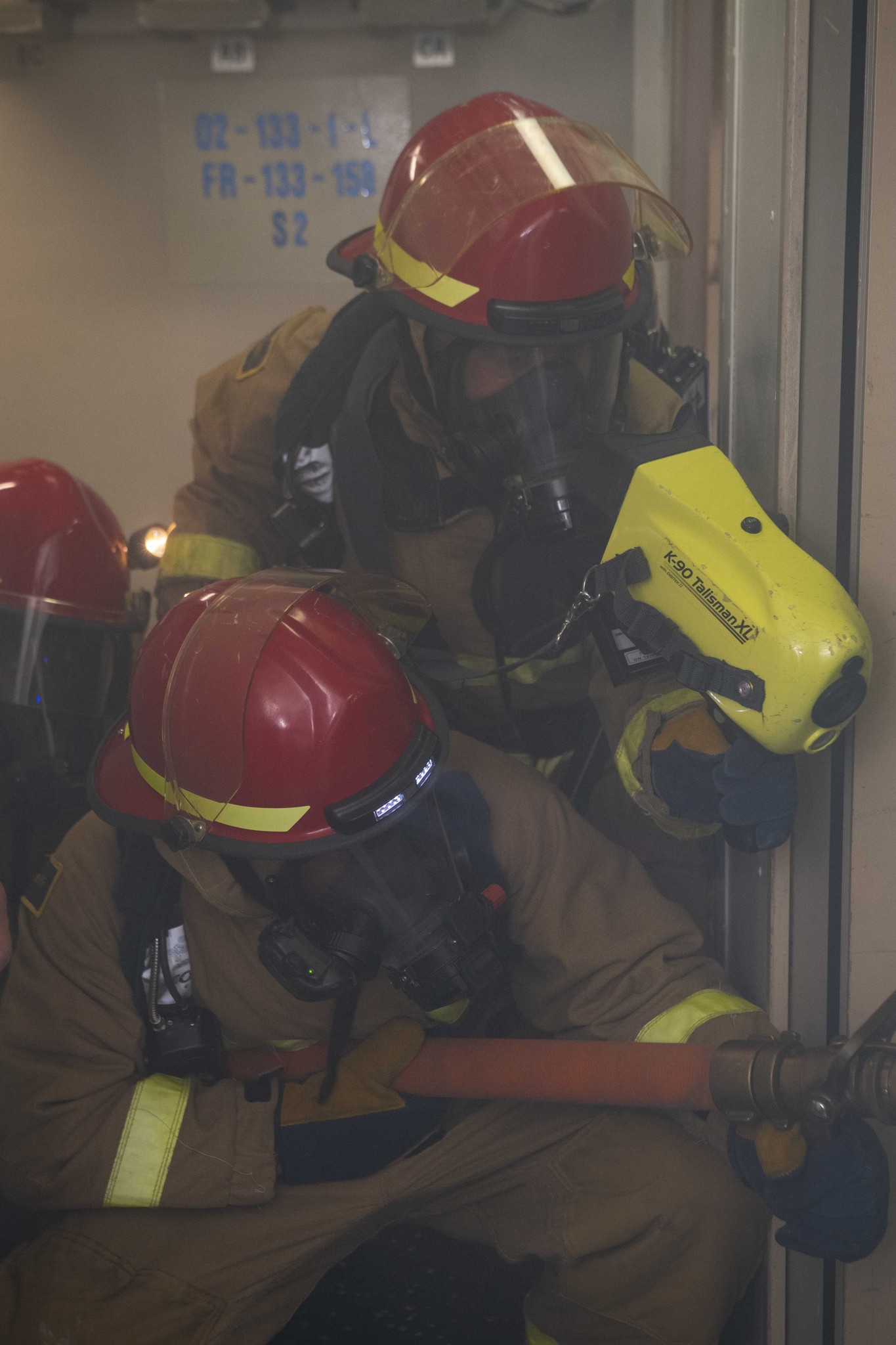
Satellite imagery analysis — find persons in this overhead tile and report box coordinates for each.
[0,562,896,1345]
[152,93,801,1084]
[0,458,133,904]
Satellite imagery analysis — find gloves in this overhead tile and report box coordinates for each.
[317,570,439,664]
[274,1014,476,1182]
[726,1112,893,1261]
[650,701,798,852]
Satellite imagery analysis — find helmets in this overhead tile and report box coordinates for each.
[93,578,449,860]
[325,92,656,345]
[0,460,133,624]
